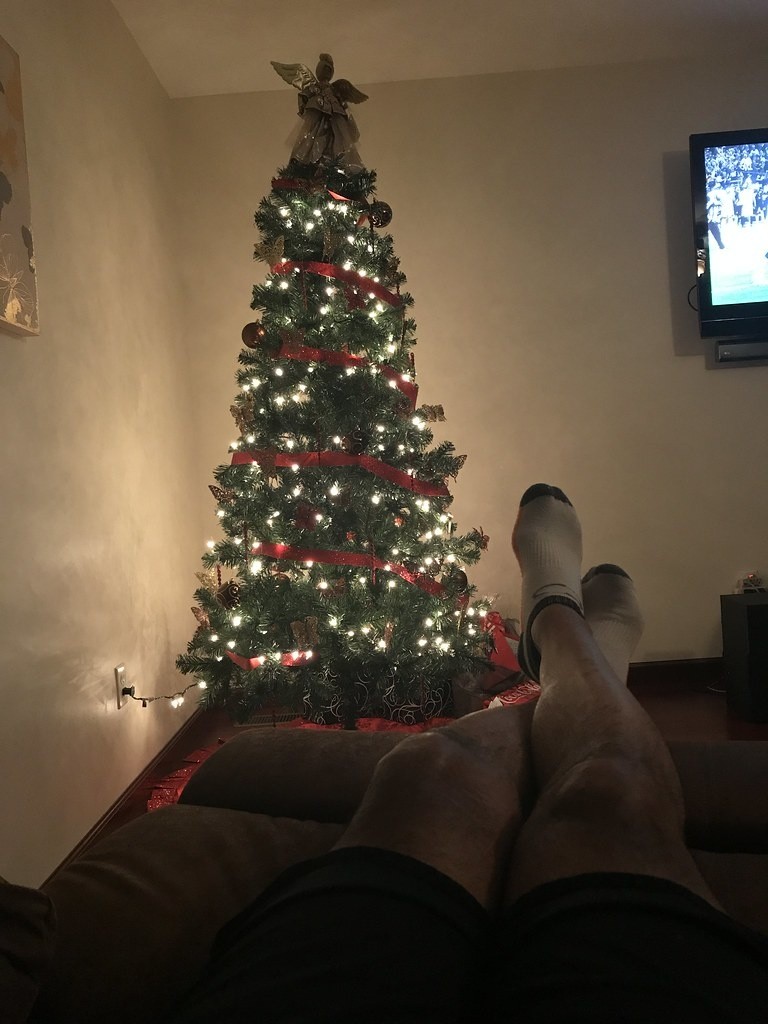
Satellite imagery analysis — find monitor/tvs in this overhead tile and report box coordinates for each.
[688,129,768,338]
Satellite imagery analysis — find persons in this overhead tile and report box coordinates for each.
[168,483,768,1024]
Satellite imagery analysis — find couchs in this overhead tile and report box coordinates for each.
[0,726,768,1023]
